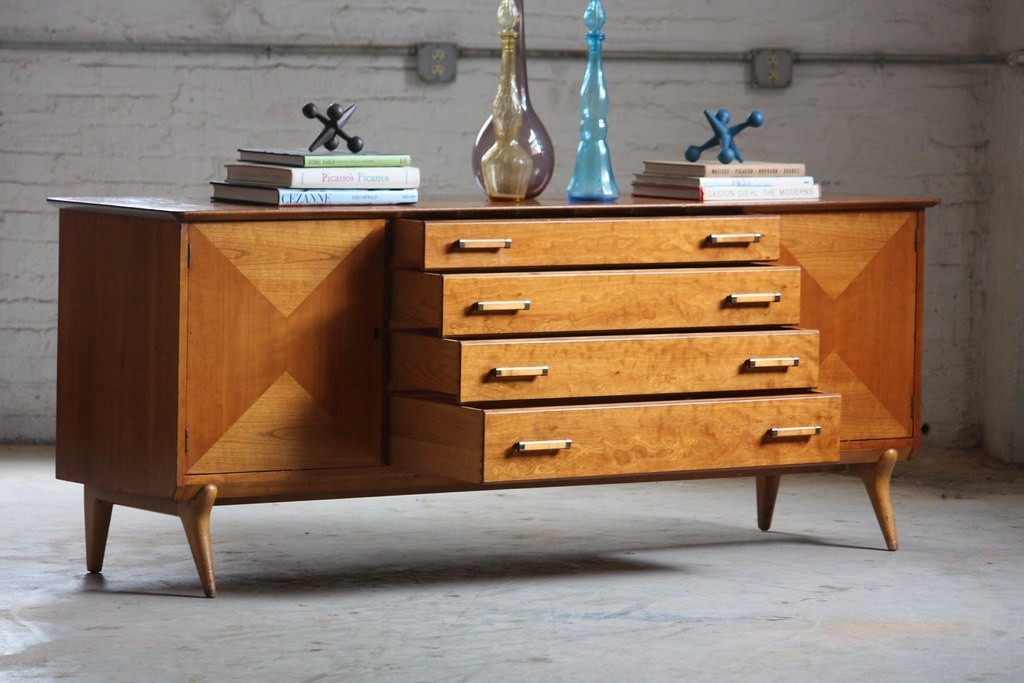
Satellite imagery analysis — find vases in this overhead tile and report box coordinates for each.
[471,0,556,199]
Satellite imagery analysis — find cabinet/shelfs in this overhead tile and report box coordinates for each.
[46,197,944,598]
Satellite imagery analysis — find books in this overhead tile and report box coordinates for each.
[631,159,819,203]
[209,148,420,208]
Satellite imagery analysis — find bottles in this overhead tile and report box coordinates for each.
[471,0,554,203]
[482,0,531,204]
[566,0,621,204]
